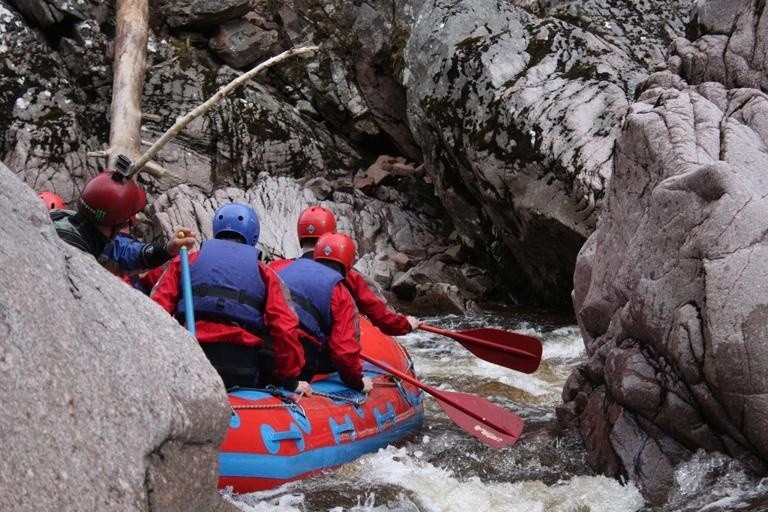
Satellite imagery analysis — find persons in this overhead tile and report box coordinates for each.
[105,213,163,296]
[266,206,424,373]
[276,232,374,393]
[151,202,312,399]
[48,172,196,273]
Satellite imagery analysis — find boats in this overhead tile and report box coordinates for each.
[218,315,426,507]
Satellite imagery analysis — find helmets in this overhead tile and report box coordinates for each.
[76,169,148,227]
[313,231,356,272]
[297,205,336,243]
[213,203,260,245]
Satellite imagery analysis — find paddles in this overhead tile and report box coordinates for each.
[420,323,542,373]
[359,352,524,451]
